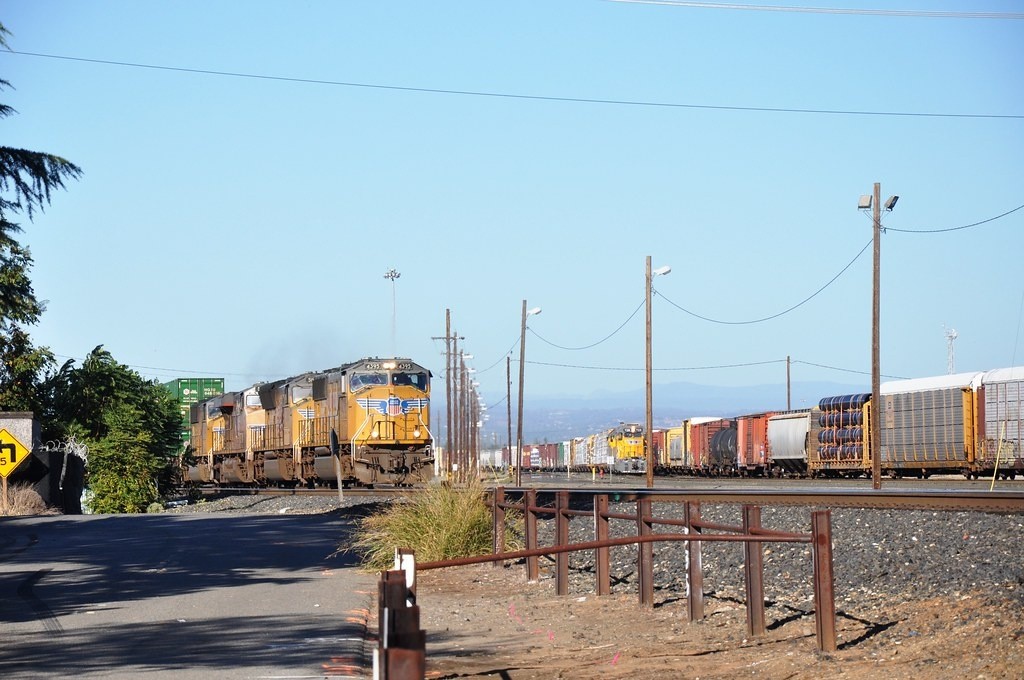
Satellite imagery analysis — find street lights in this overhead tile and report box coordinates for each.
[383,269,401,356]
[442,349,487,483]
[858,183,899,490]
[516,299,542,487]
[646,255,671,488]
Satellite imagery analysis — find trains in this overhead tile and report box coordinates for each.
[162,356,435,490]
[479,422,647,475]
[651,366,1024,480]
